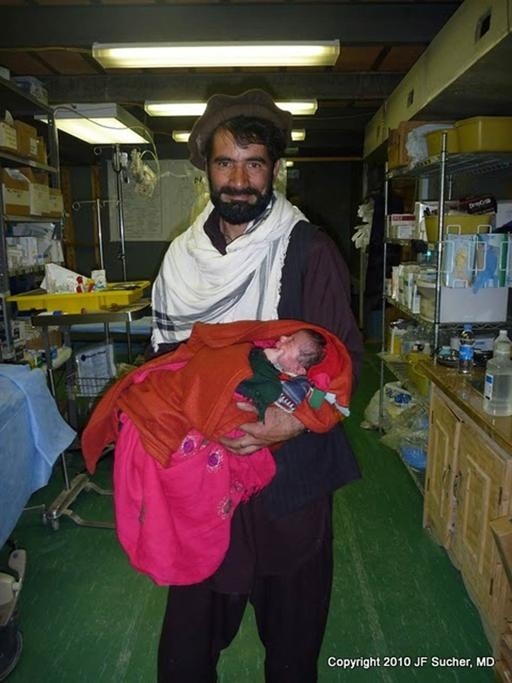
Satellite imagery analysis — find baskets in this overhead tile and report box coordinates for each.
[387,120,455,169]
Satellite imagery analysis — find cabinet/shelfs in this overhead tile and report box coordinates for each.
[418,356,512,662]
[379,132,512,495]
[0,75,73,364]
[31,298,152,530]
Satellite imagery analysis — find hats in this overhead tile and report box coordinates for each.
[188,90,294,170]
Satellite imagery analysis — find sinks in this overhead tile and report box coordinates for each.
[464,376,512,408]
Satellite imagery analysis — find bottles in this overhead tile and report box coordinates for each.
[458,324,475,374]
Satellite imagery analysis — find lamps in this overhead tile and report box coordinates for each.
[91,39,341,144]
[35,101,153,144]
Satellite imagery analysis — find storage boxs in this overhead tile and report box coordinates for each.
[422,212,491,243]
[7,279,151,315]
[0,110,66,220]
[384,115,511,169]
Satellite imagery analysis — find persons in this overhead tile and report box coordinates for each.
[144,88,363,683]
